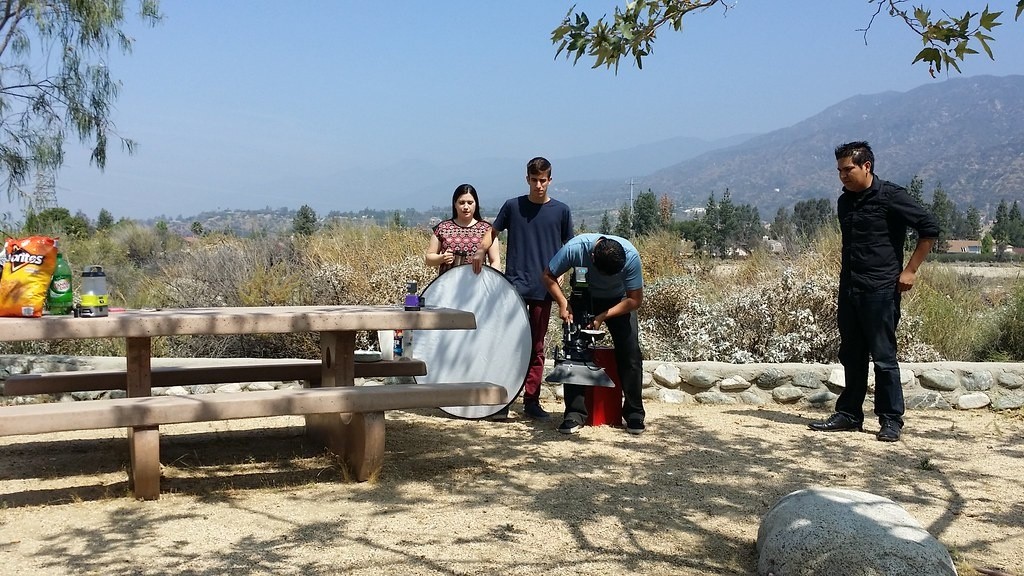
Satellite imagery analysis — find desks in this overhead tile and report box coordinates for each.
[0,302,478,502]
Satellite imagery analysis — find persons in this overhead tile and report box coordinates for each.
[426,183,501,271]
[541,233,646,435]
[807,141,940,442]
[472,157,574,419]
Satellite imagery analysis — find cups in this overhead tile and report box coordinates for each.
[378,330,394,360]
[447,250,468,269]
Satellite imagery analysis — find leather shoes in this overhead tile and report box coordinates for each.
[808,411,863,432]
[877,418,900,442]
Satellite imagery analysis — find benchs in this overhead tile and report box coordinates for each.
[0,381,509,497]
[0,357,428,397]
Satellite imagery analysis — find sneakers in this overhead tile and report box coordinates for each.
[558,419,582,433]
[491,407,508,420]
[626,420,645,433]
[524,398,551,421]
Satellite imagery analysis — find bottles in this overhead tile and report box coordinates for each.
[46,253,73,315]
[393,329,404,358]
